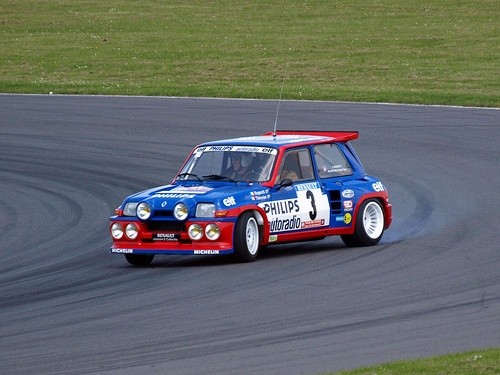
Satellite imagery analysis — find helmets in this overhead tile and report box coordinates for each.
[226,151,253,168]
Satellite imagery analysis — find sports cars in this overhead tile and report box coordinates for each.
[108,127,396,267]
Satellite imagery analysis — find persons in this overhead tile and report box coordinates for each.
[258,168,298,182]
[222,151,265,182]
